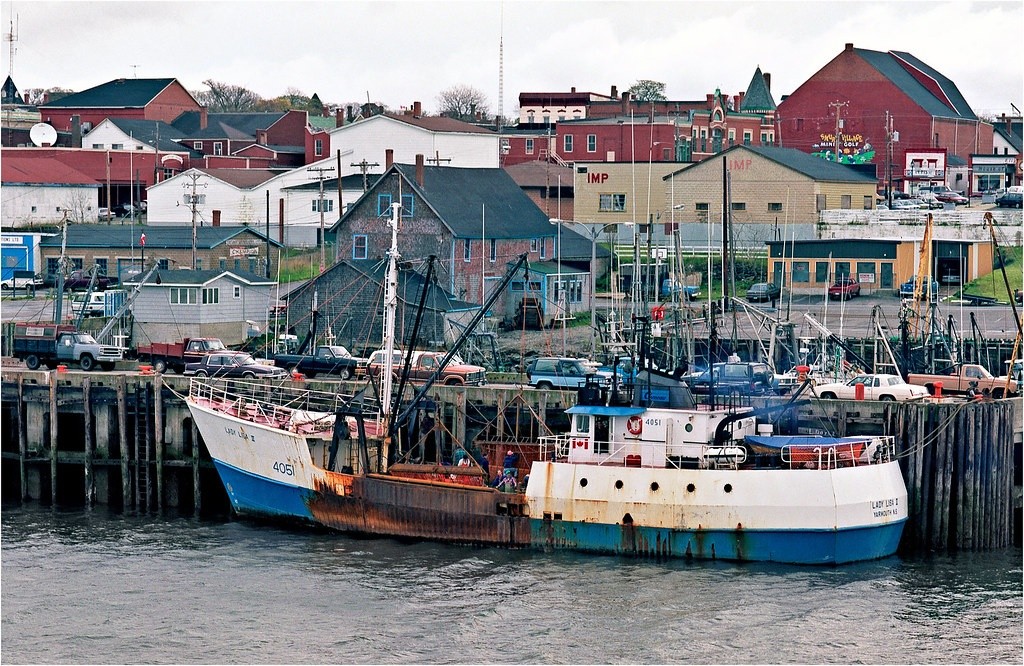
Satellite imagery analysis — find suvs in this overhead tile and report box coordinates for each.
[2,275,45,291]
[525,354,625,391]
[682,359,775,398]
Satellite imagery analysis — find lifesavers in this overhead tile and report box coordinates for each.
[627,416,642,434]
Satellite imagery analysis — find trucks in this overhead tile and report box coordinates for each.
[658,279,701,302]
[595,357,642,389]
[907,364,1017,399]
[136,337,228,375]
[181,350,287,379]
[13,320,125,371]
[274,344,369,380]
[828,278,861,301]
[365,348,488,388]
[900,275,941,300]
[57,270,109,294]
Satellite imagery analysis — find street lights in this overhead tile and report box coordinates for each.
[548,217,637,359]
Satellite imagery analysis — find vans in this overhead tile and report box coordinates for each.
[995,193,1023,208]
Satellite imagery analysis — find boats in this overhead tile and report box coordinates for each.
[161,199,912,563]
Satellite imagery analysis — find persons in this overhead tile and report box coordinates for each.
[502,450,518,477]
[479,452,489,473]
[492,469,505,491]
[496,470,518,493]
[457,455,473,467]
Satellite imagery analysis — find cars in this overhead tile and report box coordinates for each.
[772,370,799,396]
[809,372,932,402]
[942,268,961,284]
[40,273,59,287]
[875,186,1004,213]
[133,201,147,214]
[745,282,780,302]
[98,207,116,222]
[110,203,139,219]
